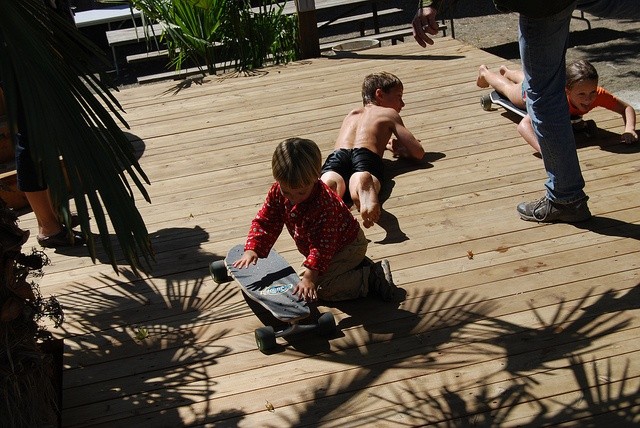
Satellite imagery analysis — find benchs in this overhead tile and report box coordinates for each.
[74,0,172,28]
[105,0,369,74]
[126,7,405,79]
[136,21,447,85]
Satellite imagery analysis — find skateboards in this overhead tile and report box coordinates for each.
[210,243,336,352]
[480,90,597,137]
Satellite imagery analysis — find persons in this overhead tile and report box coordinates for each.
[319,70,424,229]
[475,59,638,159]
[411,0,602,223]
[230,138,394,309]
[0,1,80,246]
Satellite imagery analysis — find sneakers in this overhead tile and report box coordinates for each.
[517,197,591,224]
[373,259,394,297]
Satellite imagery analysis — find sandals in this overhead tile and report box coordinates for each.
[36,230,100,247]
